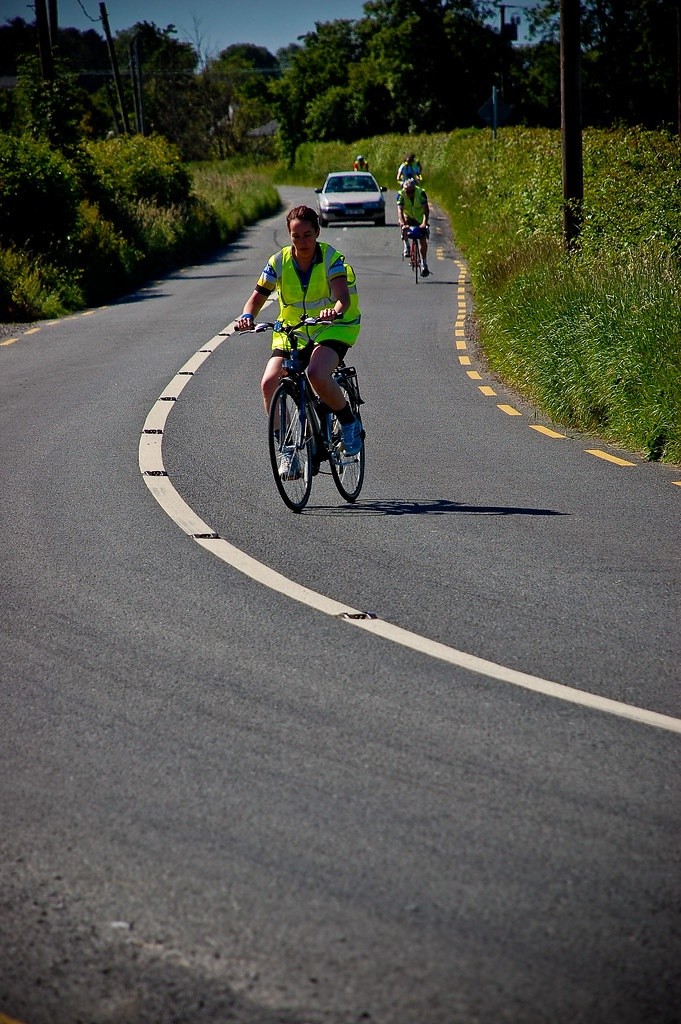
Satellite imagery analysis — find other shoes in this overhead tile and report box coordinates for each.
[422,264,428,274]
[404,250,410,257]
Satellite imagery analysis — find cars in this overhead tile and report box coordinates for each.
[314,172,387,228]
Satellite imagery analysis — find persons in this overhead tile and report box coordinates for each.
[396,179,429,277]
[397,156,420,187]
[354,154,368,172]
[235,205,362,482]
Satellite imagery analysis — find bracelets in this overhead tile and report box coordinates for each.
[241,314,254,320]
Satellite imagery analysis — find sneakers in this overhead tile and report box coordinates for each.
[341,414,363,457]
[278,453,301,478]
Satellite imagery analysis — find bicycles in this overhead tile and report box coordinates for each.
[233,312,366,512]
[399,223,430,283]
